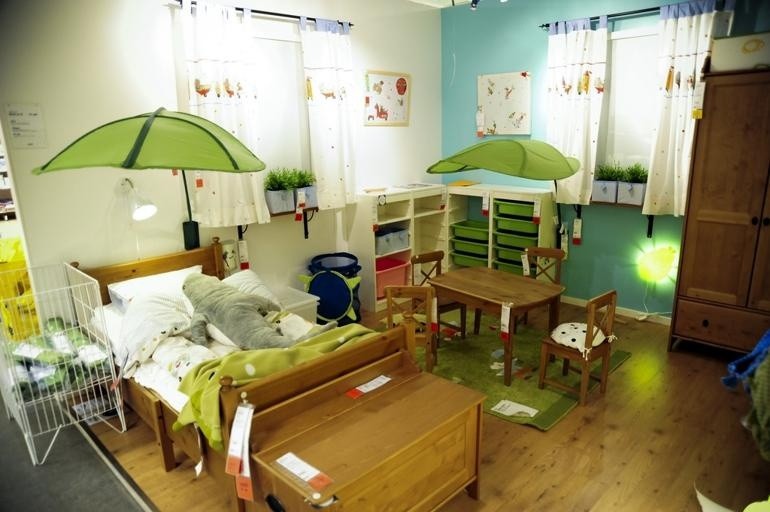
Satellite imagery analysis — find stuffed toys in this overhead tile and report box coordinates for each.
[180,268,339,351]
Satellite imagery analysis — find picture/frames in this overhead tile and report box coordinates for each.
[475,69,533,137]
[364,69,410,128]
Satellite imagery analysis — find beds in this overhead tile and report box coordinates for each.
[70,236,416,511]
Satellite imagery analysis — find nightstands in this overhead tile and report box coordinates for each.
[264,278,322,326]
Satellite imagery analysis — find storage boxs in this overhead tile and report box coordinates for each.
[374,227,410,258]
[374,257,412,300]
[451,199,538,278]
[706,30,770,73]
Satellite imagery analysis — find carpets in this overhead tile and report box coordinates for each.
[0,392,156,510]
[424,309,633,433]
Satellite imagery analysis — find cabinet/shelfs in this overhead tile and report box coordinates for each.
[447,183,559,287]
[244,351,490,511]
[666,64,770,359]
[343,182,469,315]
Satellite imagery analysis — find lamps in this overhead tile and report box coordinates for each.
[116,176,158,225]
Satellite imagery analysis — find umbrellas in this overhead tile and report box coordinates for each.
[38,105,268,253]
[426,138,584,253]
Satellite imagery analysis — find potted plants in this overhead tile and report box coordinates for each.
[264,168,318,216]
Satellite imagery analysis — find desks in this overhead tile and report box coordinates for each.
[427,264,566,389]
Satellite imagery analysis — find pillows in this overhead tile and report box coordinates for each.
[103,263,206,314]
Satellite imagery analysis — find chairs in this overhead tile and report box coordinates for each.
[408,250,468,340]
[514,246,566,332]
[535,290,618,406]
[384,284,438,375]
